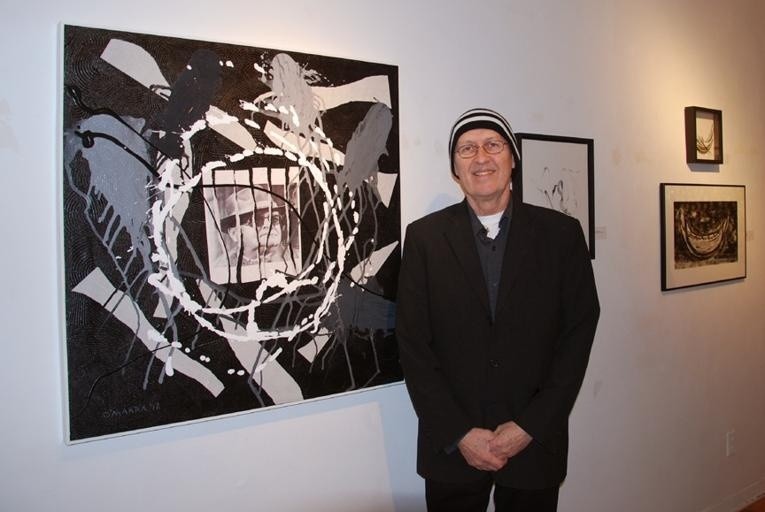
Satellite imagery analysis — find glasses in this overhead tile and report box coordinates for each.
[454,140,509,159]
[244,214,283,228]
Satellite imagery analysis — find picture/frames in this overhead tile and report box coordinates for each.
[57,22,404,446]
[659,182,747,292]
[684,106,723,164]
[512,132,595,260]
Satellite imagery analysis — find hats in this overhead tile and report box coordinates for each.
[448,107,522,185]
[219,185,286,234]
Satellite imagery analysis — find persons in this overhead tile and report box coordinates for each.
[392,107,603,512]
